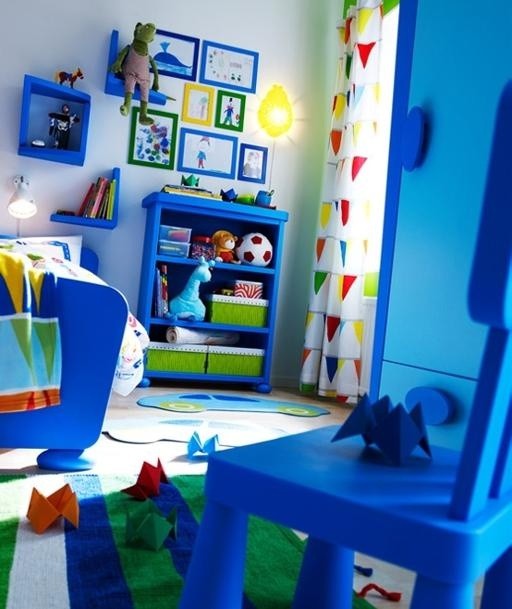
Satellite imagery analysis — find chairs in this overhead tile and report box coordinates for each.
[172,74,511,608]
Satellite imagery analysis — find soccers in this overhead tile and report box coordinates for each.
[235,232,273,267]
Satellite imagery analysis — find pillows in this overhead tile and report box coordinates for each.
[0,231,87,268]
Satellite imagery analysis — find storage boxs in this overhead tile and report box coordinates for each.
[158,238,193,259]
[145,336,207,374]
[202,290,271,330]
[207,342,267,377]
[159,223,195,243]
[233,276,264,299]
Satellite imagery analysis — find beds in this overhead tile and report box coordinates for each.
[0,233,153,478]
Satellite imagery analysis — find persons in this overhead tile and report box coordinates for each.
[49,102,80,149]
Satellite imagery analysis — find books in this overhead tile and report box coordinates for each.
[75,177,117,221]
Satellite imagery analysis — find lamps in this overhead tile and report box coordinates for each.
[8,171,39,238]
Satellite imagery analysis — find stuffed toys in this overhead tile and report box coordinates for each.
[212,229,242,264]
[166,256,215,322]
[111,24,160,126]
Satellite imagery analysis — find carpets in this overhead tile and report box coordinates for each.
[0,469,378,608]
[134,389,332,421]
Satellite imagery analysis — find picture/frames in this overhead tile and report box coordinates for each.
[127,28,270,186]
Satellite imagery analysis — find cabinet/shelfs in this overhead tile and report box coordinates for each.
[131,187,292,394]
[365,1,511,460]
[15,27,169,231]
